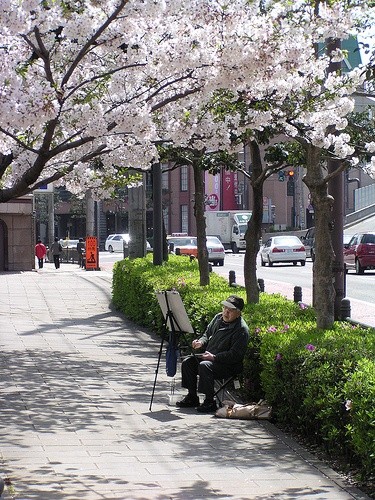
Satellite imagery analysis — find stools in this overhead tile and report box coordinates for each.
[211,376,239,408]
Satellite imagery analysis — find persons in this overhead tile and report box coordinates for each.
[50,237,62,269]
[35,239,46,268]
[176,295,250,412]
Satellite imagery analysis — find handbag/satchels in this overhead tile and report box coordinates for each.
[215,399,273,420]
[59,243,62,252]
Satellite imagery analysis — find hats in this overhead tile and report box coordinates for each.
[220,295,244,312]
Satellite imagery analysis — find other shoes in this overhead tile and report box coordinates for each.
[175,393,201,408]
[197,399,218,413]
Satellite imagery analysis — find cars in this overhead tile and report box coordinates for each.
[343,231,375,275]
[104,233,152,253]
[166,232,198,259]
[206,235,225,266]
[259,235,307,267]
[299,225,316,262]
[45,239,80,263]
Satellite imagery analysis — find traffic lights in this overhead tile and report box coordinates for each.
[278,170,295,176]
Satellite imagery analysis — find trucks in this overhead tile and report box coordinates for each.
[204,209,253,254]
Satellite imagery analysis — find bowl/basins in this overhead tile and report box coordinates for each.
[167,393,184,406]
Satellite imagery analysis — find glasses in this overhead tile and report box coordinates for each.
[222,306,232,312]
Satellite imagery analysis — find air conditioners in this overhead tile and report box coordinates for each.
[235,194,242,204]
